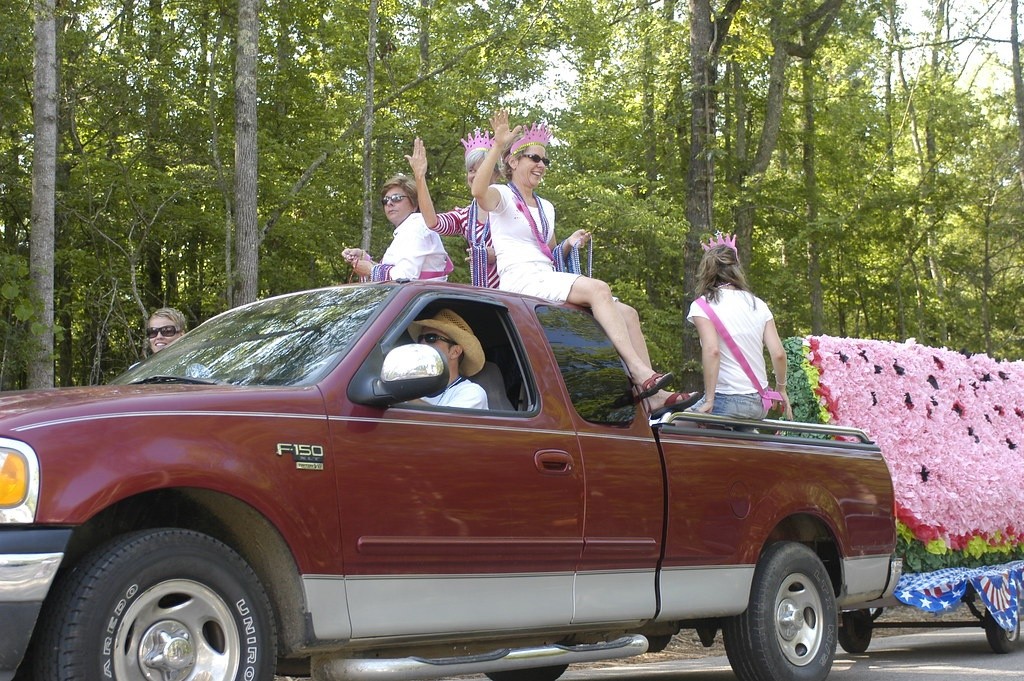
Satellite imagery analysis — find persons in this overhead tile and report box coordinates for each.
[404,126,501,289]
[671,230,794,435]
[128,308,212,379]
[340,174,454,283]
[471,108,700,420]
[407,308,489,410]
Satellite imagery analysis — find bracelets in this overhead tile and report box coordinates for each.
[704,397,714,402]
[775,374,787,386]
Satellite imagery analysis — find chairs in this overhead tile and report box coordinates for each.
[469,360,517,410]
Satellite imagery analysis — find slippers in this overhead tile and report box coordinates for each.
[640,373,673,399]
[651,392,699,420]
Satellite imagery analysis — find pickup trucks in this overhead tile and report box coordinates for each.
[0,279,903,681]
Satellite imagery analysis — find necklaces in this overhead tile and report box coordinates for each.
[467,195,490,249]
[349,250,395,283]
[507,181,549,243]
[470,244,488,287]
[552,235,592,278]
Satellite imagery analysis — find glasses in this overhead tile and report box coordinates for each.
[382,194,409,205]
[519,154,550,166]
[146,325,181,338]
[418,333,457,346]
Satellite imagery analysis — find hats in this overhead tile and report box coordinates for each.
[407,308,485,377]
